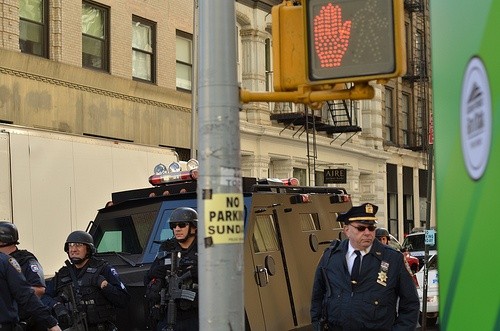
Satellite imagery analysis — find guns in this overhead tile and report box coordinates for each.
[161,250,196,331]
[57,282,89,331]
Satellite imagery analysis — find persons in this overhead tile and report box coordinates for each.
[41,231,132,331]
[0,251,63,331]
[375,228,414,278]
[310,203,420,331]
[148,207,198,331]
[0,221,47,298]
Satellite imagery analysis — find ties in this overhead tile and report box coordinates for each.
[350,250,362,292]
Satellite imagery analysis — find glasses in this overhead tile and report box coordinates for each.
[68,242,86,248]
[171,222,189,229]
[348,223,377,232]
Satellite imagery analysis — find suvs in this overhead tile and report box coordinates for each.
[389,230,439,313]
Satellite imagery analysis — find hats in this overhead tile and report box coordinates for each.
[336,203,379,225]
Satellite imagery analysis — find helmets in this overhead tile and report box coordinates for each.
[374,228,390,241]
[170,207,198,223]
[64,231,94,251]
[0,221,19,244]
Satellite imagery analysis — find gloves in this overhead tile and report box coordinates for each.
[151,265,168,280]
[96,275,106,287]
[54,303,72,329]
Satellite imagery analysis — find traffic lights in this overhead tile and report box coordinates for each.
[302,1,408,87]
[269,3,341,93]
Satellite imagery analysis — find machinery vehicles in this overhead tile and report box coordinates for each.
[44,160,355,330]
